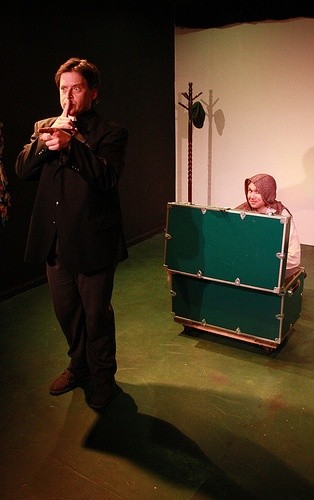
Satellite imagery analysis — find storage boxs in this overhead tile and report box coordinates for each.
[163,201,306,349]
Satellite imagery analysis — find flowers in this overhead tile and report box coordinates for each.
[0,122,15,232]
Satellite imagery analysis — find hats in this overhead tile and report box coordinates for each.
[190,102,205,129]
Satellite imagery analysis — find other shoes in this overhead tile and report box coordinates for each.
[90,393,112,408]
[49,370,78,394]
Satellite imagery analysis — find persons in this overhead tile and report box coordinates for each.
[233,174,301,270]
[15,56,130,408]
